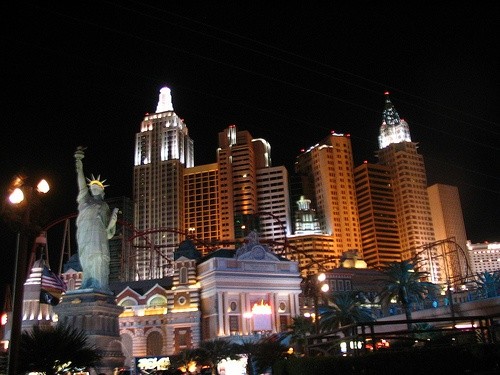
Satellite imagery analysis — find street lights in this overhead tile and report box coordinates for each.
[311,273,330,333]
[3,174,49,374]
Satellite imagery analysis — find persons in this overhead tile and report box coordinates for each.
[75,145,120,292]
